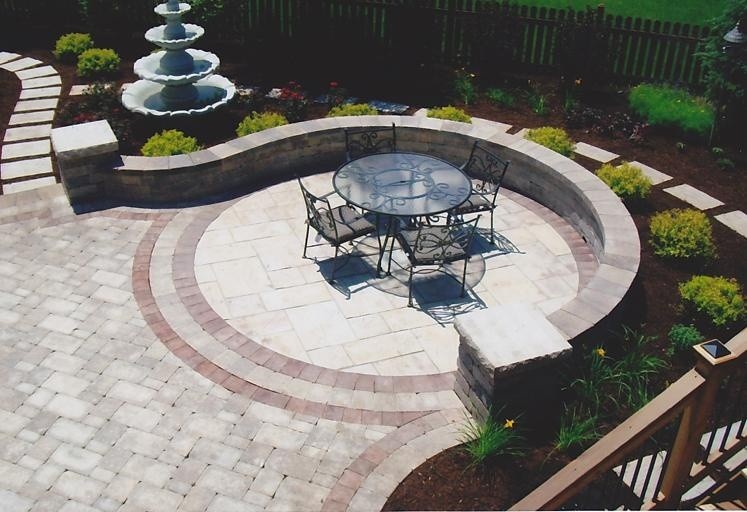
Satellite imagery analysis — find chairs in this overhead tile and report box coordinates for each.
[298,175,383,285]
[345,123,396,161]
[386,214,482,307]
[446,138,511,244]
